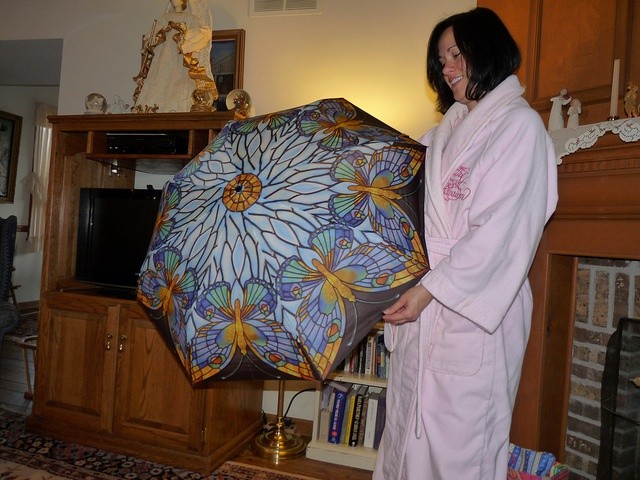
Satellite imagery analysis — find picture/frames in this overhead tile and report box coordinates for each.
[198,28,246,110]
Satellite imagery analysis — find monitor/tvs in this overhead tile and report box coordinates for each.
[75,187,162,300]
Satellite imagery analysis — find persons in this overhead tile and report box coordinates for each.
[373,6,558,479]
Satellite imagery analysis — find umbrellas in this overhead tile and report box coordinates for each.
[136,96,432,387]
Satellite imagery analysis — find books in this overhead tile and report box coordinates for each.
[318,382,387,451]
[334,331,391,380]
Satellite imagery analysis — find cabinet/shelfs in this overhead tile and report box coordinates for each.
[22,114,267,475]
[304,322,407,472]
[471,0,639,480]
